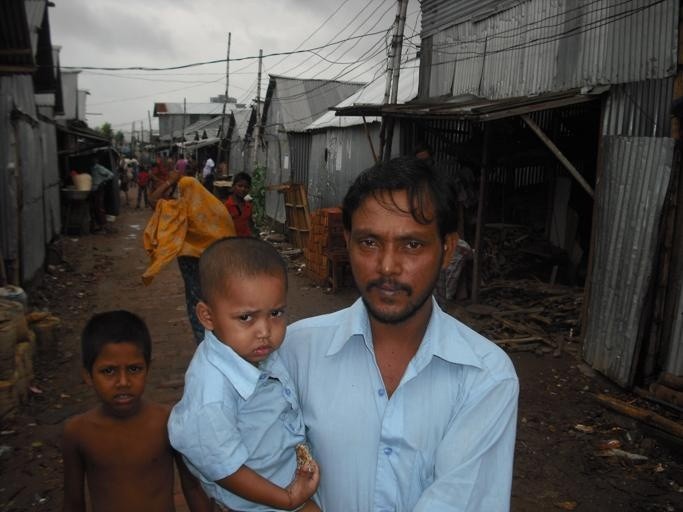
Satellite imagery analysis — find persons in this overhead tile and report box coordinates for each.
[156,176,238,347]
[220,172,258,239]
[163,235,320,512]
[58,309,215,512]
[272,152,521,512]
[83,151,231,235]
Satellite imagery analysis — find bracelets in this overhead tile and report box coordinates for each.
[165,179,172,187]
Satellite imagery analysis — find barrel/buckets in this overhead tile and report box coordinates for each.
[78,174,92,191]
[0,285,27,380]
[31,316,62,354]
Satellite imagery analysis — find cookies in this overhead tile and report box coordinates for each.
[294,444,315,472]
